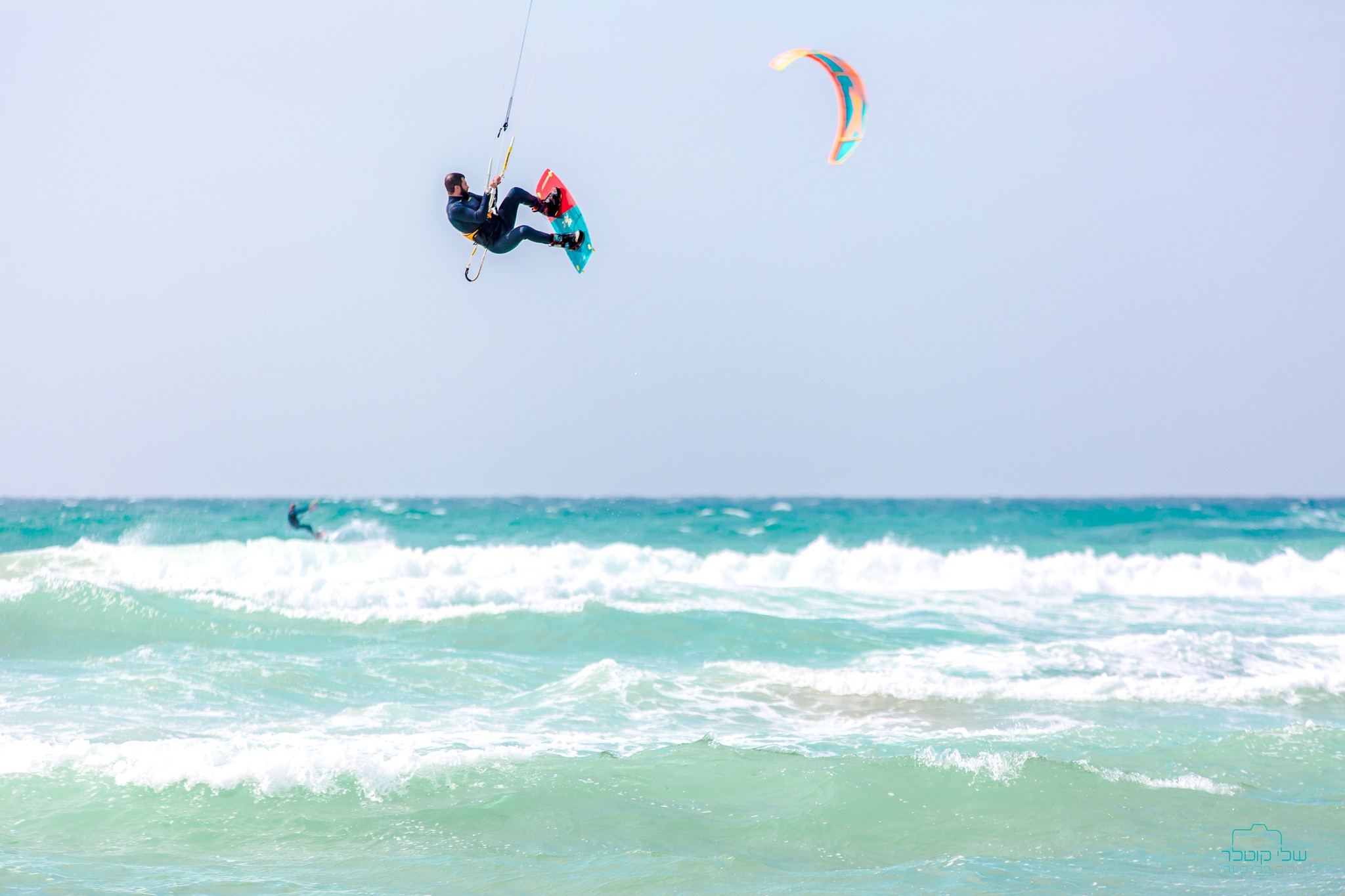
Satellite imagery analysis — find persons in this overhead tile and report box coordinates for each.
[287,502,317,539]
[444,173,584,254]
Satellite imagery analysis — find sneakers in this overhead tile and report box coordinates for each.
[533,187,562,217]
[549,230,585,251]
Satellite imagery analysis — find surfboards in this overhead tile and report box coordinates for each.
[536,168,596,274]
